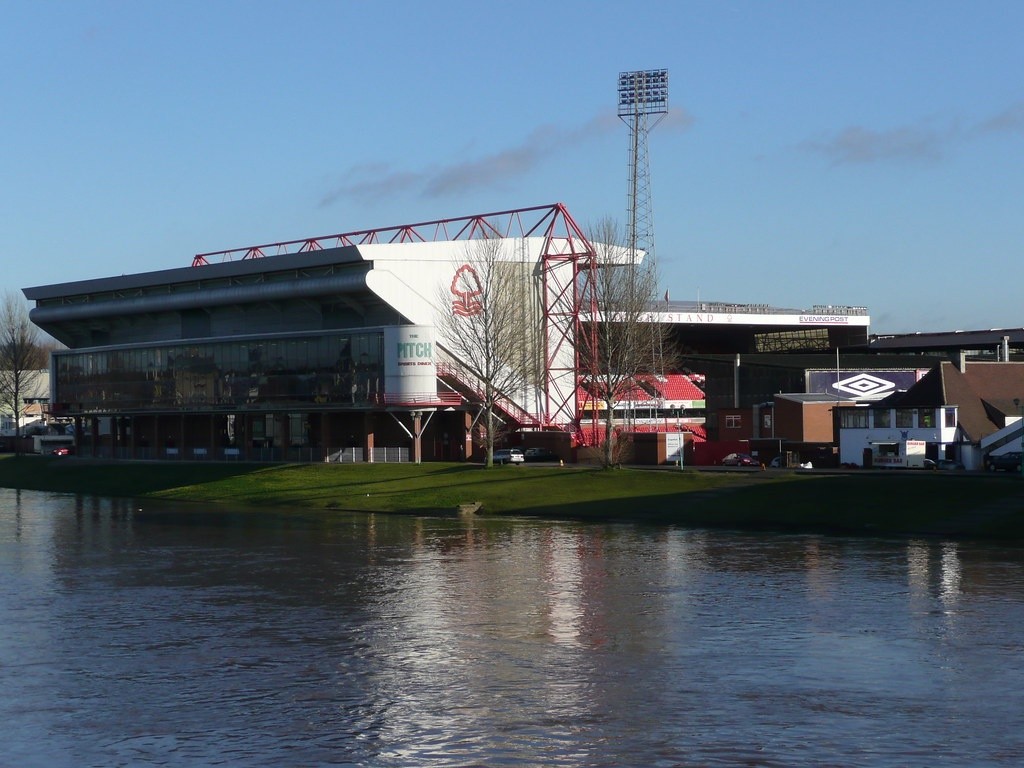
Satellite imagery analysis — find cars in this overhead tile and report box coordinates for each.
[720,453,760,467]
[987,452,1024,473]
[924,459,938,470]
[525,447,559,461]
[937,459,965,471]
[771,457,785,467]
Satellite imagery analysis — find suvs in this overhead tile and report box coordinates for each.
[485,448,524,465]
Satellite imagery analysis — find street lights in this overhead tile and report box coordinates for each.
[615,67,671,455]
